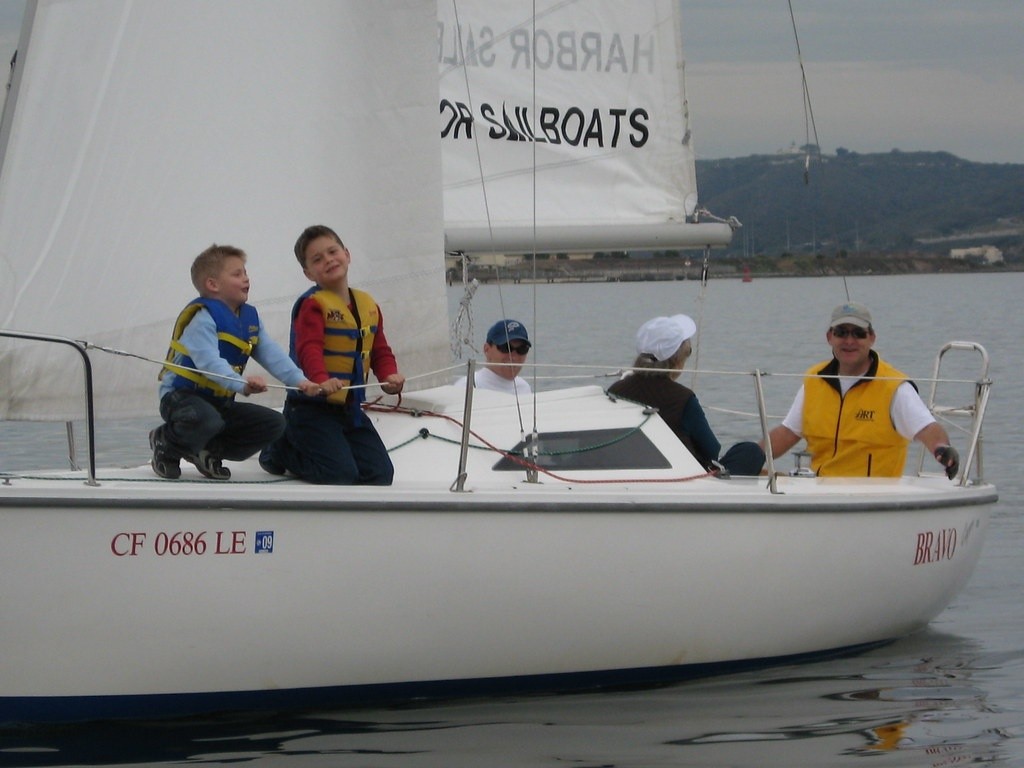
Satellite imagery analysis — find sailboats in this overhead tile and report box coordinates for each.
[1,1,1005,719]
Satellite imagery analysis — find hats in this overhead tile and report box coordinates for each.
[636,314,695,361]
[487,319,531,347]
[829,303,873,328]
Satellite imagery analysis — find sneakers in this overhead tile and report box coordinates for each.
[183,440,231,479]
[148,423,181,478]
[259,441,286,474]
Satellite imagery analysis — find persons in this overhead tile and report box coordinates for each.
[259,224,405,486]
[758,301,960,480]
[149,245,320,479]
[452,319,533,396]
[605,314,766,477]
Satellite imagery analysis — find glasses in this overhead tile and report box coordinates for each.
[496,343,530,355]
[833,326,870,339]
